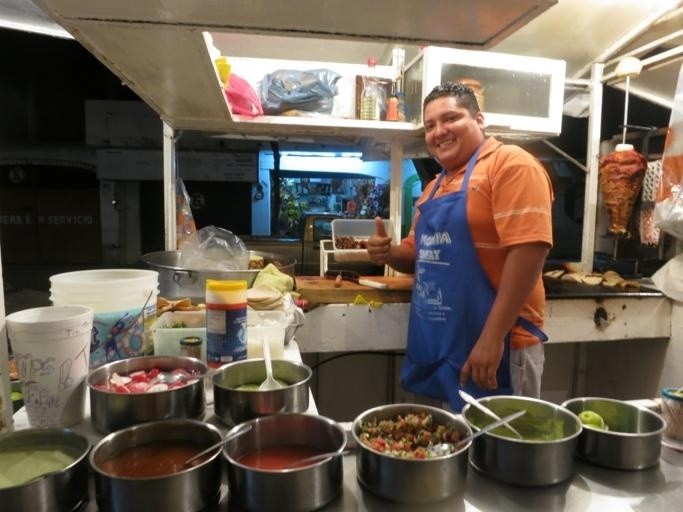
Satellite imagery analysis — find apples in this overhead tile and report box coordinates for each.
[579,410,604,431]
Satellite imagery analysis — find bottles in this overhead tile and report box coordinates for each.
[384,89,408,124]
[359,52,381,124]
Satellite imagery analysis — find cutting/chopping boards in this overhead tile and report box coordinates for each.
[292,273,414,302]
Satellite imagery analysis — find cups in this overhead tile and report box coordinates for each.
[4,303,94,429]
[662,387,683,441]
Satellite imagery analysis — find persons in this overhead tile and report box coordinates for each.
[366,83,554,418]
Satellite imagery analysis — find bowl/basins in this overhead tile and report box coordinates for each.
[228,414,348,510]
[84,355,210,437]
[456,393,584,488]
[555,395,666,471]
[1,428,92,511]
[213,357,315,430]
[352,393,474,504]
[90,416,226,511]
